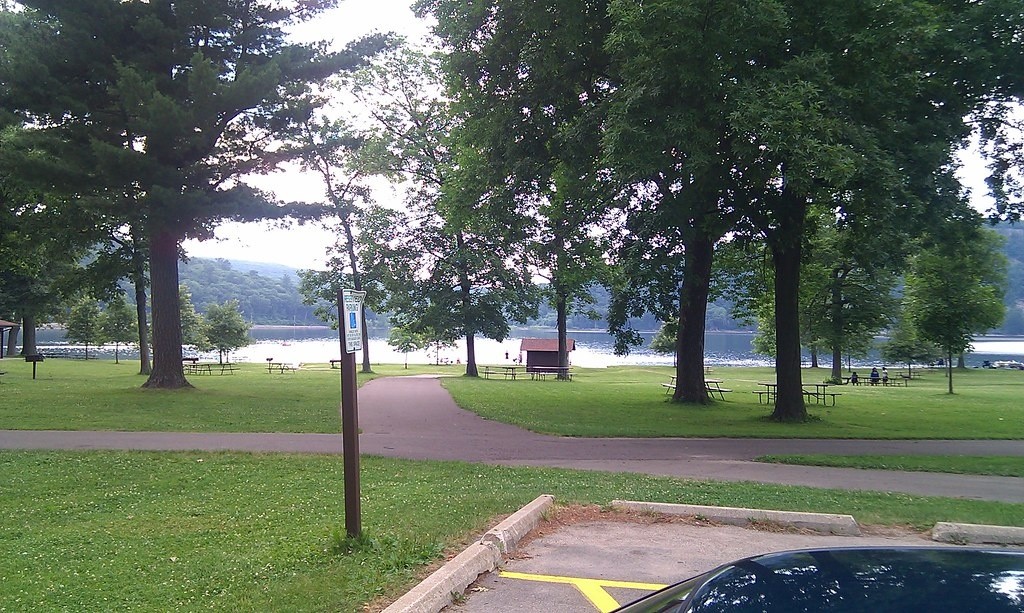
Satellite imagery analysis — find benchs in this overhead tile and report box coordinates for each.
[183,367,239,371]
[42,354,70,357]
[705,370,714,372]
[752,390,842,397]
[479,371,575,375]
[928,363,946,369]
[72,357,99,359]
[661,383,733,393]
[893,374,922,376]
[848,381,903,384]
[265,366,300,370]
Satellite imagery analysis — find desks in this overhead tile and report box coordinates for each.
[850,376,902,388]
[758,383,829,404]
[894,371,920,380]
[666,375,725,401]
[270,362,295,374]
[42,352,71,358]
[73,354,96,360]
[480,366,573,381]
[188,362,235,376]
[704,366,713,374]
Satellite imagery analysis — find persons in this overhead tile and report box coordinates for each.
[851,372,858,386]
[882,367,888,386]
[870,367,879,386]
[518,352,522,363]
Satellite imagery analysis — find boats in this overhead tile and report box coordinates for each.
[993,359,1024,370]
